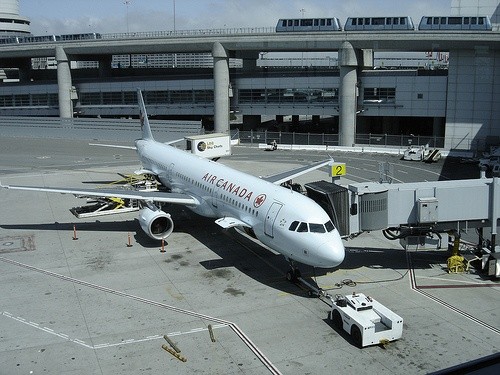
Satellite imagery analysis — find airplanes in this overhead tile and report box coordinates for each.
[0,89,346,280]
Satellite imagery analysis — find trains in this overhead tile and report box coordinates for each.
[275,15,492,32]
[0,33,102,44]
